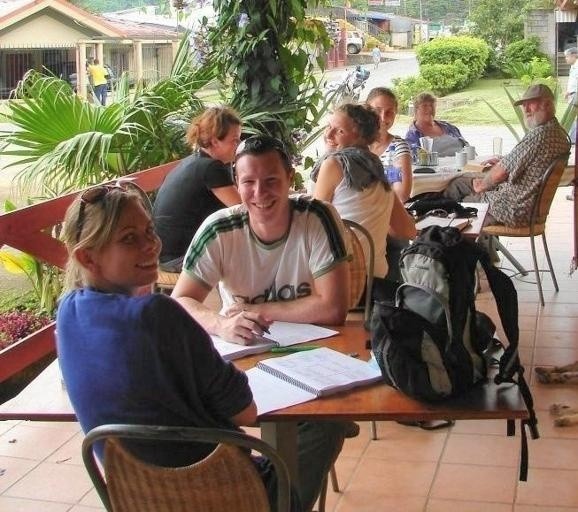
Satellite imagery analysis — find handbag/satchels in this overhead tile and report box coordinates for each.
[405,192,478,218]
[104,65,113,80]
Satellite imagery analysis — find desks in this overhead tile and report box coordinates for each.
[1,311,530,512]
[400,202,528,295]
[410,156,500,195]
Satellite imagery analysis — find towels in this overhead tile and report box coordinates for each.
[419,135,433,153]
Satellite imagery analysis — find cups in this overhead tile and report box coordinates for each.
[420,136,433,152]
[493,137,502,155]
[420,153,428,166]
[456,152,467,167]
[464,147,475,159]
[429,152,438,165]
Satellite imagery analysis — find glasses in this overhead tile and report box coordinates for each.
[76,181,125,243]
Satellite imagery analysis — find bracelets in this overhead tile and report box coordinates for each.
[483,175,495,190]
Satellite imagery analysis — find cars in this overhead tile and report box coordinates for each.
[323,28,364,56]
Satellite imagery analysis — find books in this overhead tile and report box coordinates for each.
[210,320,340,360]
[243,347,383,416]
[415,215,473,234]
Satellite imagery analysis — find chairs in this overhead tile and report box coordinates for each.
[481,153,572,307]
[82,424,339,512]
[343,219,378,440]
[150,268,182,296]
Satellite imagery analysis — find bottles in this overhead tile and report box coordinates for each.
[383,145,402,186]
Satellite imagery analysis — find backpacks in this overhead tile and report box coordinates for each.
[371,225,540,481]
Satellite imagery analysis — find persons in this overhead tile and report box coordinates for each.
[169,136,354,512]
[367,87,413,205]
[442,84,570,226]
[565,47,578,145]
[371,45,381,71]
[87,59,107,106]
[55,178,301,512]
[151,107,245,273]
[405,92,467,162]
[312,104,417,307]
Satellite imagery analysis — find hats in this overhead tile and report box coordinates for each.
[514,84,552,106]
[231,135,289,180]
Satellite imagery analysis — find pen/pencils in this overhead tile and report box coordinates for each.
[271,345,320,353]
[242,309,271,334]
